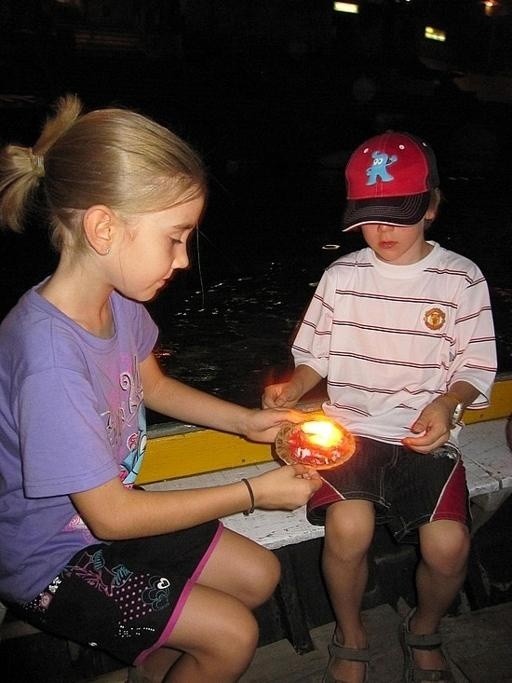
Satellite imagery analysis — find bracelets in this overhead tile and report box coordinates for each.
[239,476,256,517]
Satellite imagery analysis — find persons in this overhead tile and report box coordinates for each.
[0,89,332,681]
[262,123,501,681]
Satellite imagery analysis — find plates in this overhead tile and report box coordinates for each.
[274,416,358,471]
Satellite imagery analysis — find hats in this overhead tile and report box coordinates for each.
[340,129,439,233]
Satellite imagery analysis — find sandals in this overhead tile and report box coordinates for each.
[400,607,453,683]
[323,630,369,682]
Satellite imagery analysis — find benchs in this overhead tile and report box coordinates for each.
[2,419,508,655]
